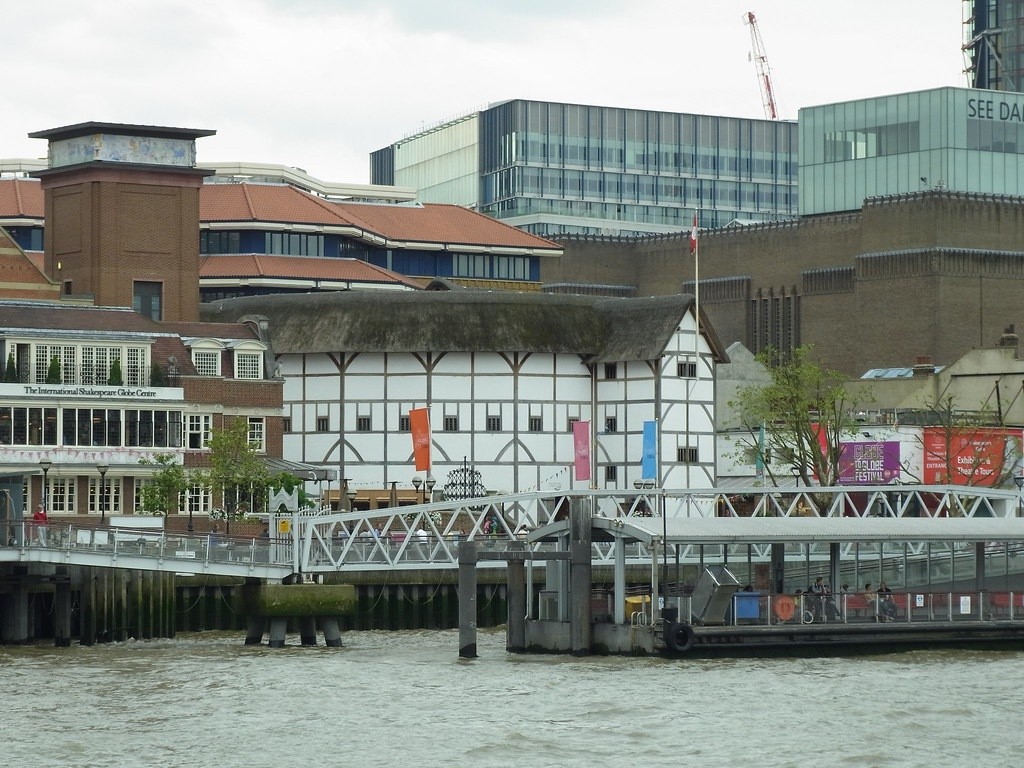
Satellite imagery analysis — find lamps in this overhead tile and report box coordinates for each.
[862,432,870,438]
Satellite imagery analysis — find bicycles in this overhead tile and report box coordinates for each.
[773,607,814,624]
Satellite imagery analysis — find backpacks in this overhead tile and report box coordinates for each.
[808,582,823,595]
[486,522,493,533]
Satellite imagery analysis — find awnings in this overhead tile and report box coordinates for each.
[249,458,337,483]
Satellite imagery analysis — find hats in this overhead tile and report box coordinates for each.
[493,517,497,520]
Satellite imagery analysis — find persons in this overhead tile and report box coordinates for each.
[724,510,731,517]
[33,505,48,548]
[260,528,270,566]
[349,516,546,546]
[743,577,895,622]
[210,524,222,563]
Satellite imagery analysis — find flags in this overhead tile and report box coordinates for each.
[689,212,696,256]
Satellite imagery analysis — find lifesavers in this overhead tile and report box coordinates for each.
[667,622,694,652]
[773,595,795,620]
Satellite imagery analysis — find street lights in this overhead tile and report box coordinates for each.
[876,493,887,517]
[633,479,655,516]
[1014,475,1024,517]
[790,467,801,517]
[347,486,359,538]
[497,488,508,533]
[412,473,436,531]
[96,459,109,524]
[772,493,781,517]
[39,457,52,525]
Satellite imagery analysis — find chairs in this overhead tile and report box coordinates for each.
[734,592,761,618]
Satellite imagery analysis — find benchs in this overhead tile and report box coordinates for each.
[991,591,1024,614]
[846,592,970,618]
